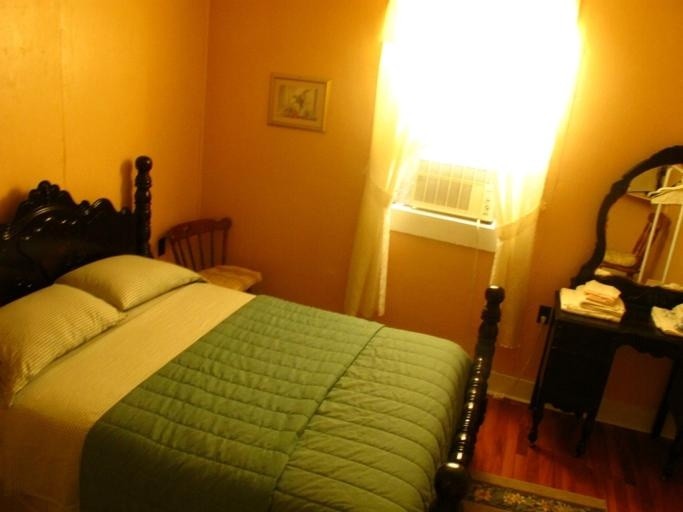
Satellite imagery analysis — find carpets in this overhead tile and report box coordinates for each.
[460,467,609,512]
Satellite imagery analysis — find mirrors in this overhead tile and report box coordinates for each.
[569,144,682,300]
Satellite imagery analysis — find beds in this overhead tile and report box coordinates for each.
[0,155,507,511]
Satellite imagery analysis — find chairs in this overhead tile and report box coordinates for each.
[165,215,263,295]
[598,208,672,289]
[647,299,681,337]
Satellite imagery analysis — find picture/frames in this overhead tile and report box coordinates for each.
[266,73,331,133]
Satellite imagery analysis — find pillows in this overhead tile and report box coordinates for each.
[0,281,129,410]
[57,253,206,314]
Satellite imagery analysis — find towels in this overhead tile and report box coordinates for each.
[559,278,624,324]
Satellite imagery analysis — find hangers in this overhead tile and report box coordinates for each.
[647,181,682,205]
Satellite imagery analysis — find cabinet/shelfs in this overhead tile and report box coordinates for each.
[527,286,682,468]
[382,173,503,362]
